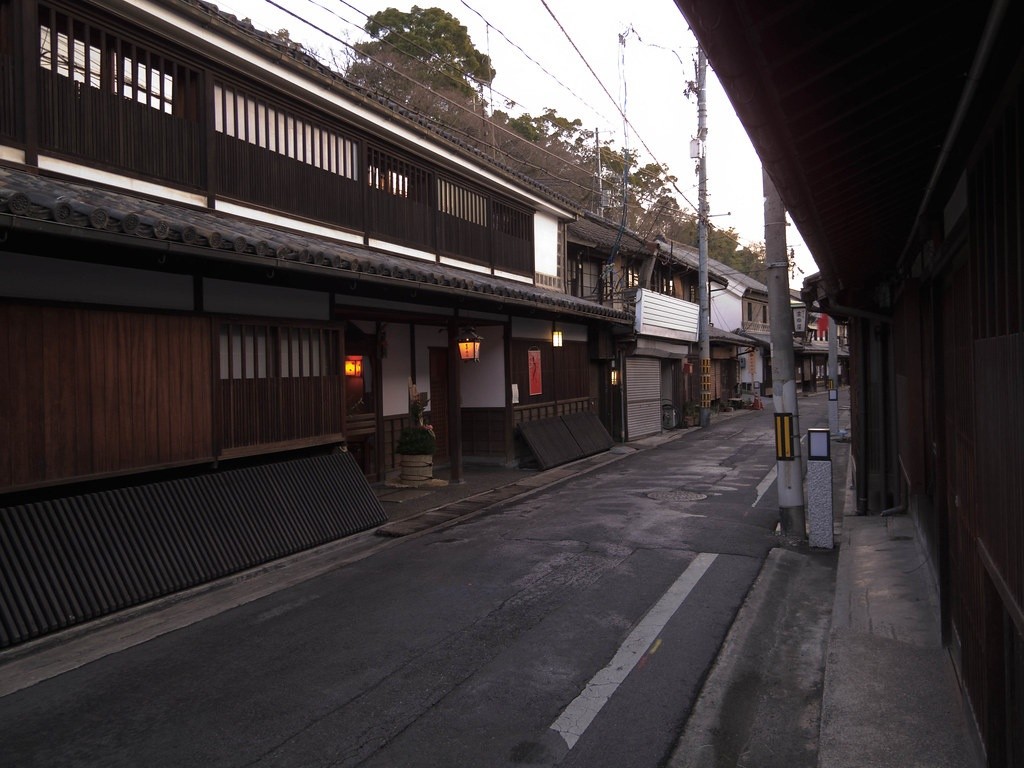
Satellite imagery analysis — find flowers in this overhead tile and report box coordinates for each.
[411,392,435,431]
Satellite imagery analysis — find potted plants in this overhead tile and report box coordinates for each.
[396,430,435,487]
[683,401,696,426]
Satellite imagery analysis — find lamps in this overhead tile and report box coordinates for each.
[344,344,365,378]
[455,325,485,364]
[611,367,618,386]
[754,381,760,389]
[551,331,563,348]
[807,427,833,461]
[828,388,838,401]
